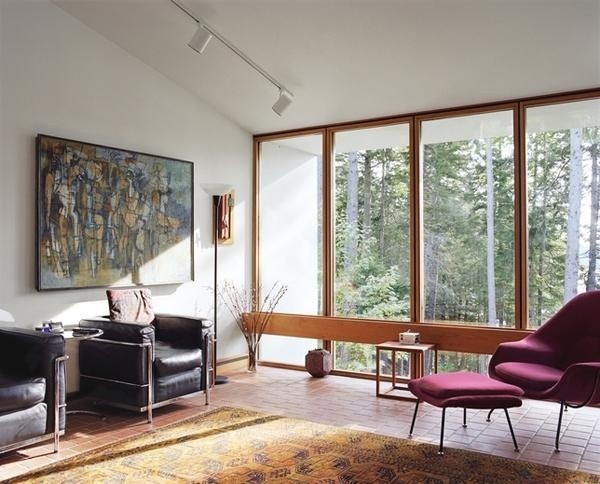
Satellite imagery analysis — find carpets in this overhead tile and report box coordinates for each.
[0,406,600,483]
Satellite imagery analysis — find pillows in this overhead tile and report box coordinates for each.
[417,372,525,399]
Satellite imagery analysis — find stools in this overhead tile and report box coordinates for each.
[409,371,524,456]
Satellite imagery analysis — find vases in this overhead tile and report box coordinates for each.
[246,343,259,373]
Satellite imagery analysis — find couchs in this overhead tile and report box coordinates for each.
[488,291,600,454]
[0,327,69,456]
[80,286,214,424]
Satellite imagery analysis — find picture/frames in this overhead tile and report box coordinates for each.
[35,132,194,291]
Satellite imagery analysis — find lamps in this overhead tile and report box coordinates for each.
[198,181,235,385]
[273,91,295,117]
[187,25,212,55]
